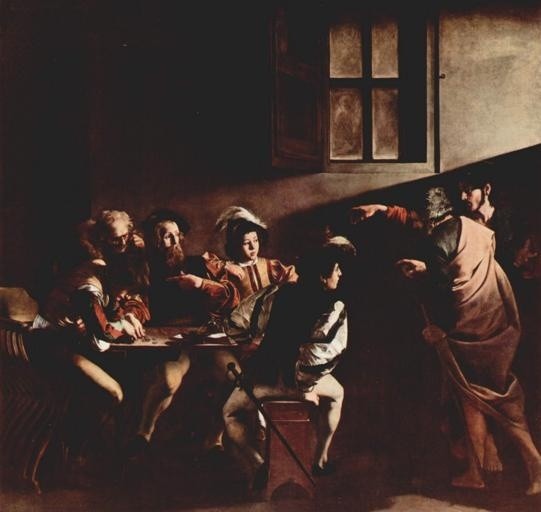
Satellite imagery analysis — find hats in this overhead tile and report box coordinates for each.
[143,211,189,242]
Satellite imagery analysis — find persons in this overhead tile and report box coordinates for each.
[27,162,541,503]
